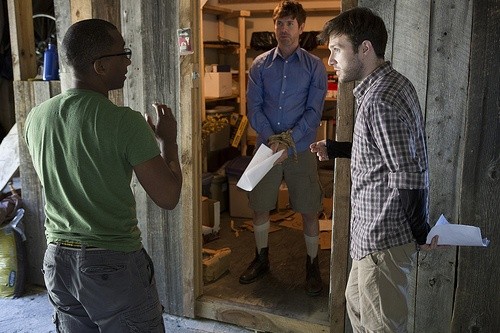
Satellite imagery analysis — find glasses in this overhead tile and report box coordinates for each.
[92,48,132,64]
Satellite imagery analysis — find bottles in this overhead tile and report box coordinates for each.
[43,37,58,80]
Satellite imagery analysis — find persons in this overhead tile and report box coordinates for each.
[239,0,329,296]
[23,18,183,332]
[310,7,450,333]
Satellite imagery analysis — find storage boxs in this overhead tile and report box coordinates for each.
[205,122,232,152]
[202,246,231,283]
[320,219,332,250]
[204,65,234,98]
[202,196,220,237]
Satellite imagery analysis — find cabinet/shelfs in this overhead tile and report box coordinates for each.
[198,0,340,187]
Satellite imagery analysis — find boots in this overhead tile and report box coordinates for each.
[239,247,269,284]
[306,253,322,296]
